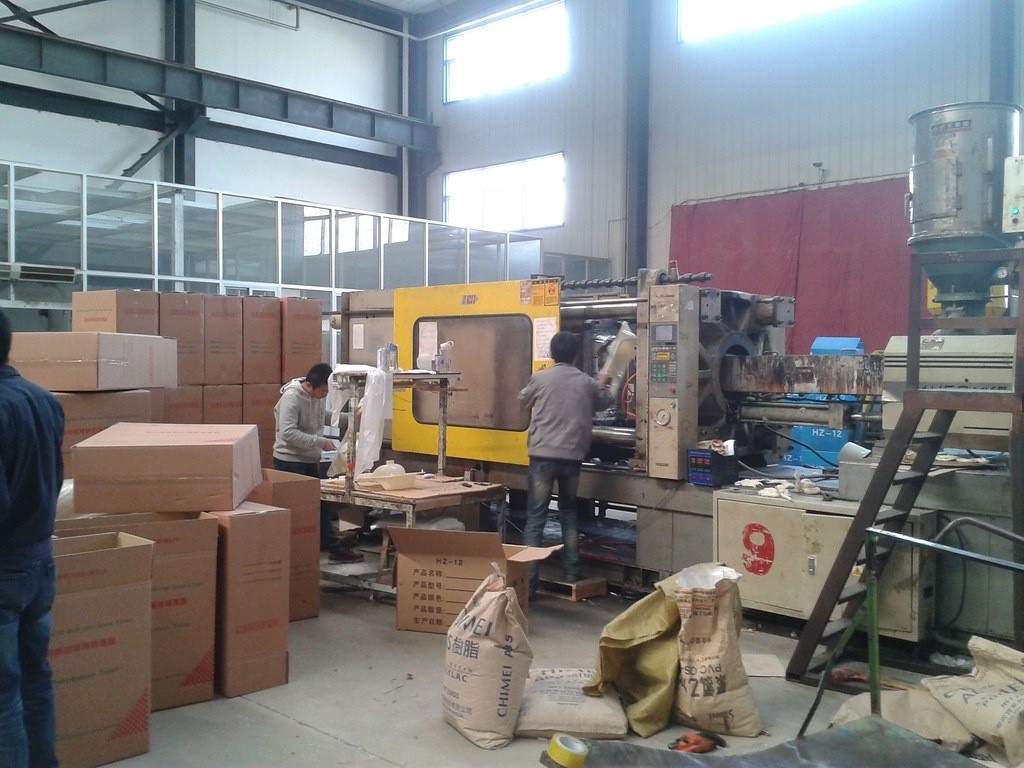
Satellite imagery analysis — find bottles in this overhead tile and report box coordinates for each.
[431,341,455,372]
[377,342,398,371]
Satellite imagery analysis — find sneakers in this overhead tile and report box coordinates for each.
[327,545,365,564]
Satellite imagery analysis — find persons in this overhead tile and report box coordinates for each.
[517,332,615,601]
[272,364,363,564]
[1,310,62,768]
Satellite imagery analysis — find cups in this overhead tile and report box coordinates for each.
[389,367,394,372]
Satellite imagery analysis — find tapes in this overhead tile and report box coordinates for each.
[547,731,588,768]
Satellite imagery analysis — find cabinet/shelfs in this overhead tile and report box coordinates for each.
[713,487,937,642]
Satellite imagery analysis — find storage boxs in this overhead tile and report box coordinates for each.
[47,468,320,768]
[8,333,178,392]
[387,526,566,635]
[71,291,324,470]
[49,389,164,479]
[71,421,264,512]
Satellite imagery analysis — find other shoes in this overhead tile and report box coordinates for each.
[558,551,580,563]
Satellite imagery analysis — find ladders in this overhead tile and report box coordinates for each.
[786,409,958,681]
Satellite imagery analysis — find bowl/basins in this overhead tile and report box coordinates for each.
[376,471,427,490]
